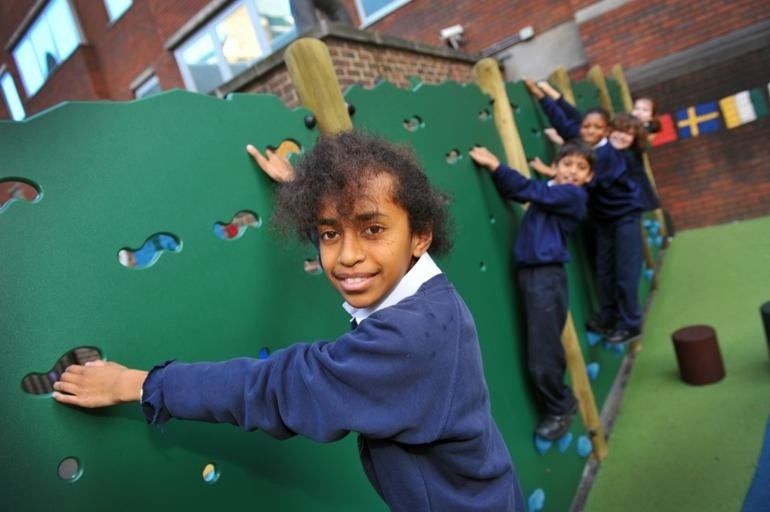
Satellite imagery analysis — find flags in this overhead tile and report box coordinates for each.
[716,86,770,130]
[674,100,722,141]
[646,114,678,148]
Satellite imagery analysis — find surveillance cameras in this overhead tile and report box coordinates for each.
[439,24,465,40]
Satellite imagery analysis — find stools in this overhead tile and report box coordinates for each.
[672,326,725,385]
[760,301,770,346]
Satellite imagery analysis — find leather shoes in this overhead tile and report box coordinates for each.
[535,401,578,440]
[586,318,642,343]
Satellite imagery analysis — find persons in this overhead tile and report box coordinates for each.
[468,140,601,441]
[627,94,657,152]
[521,71,651,346]
[528,110,662,214]
[50,127,529,512]
